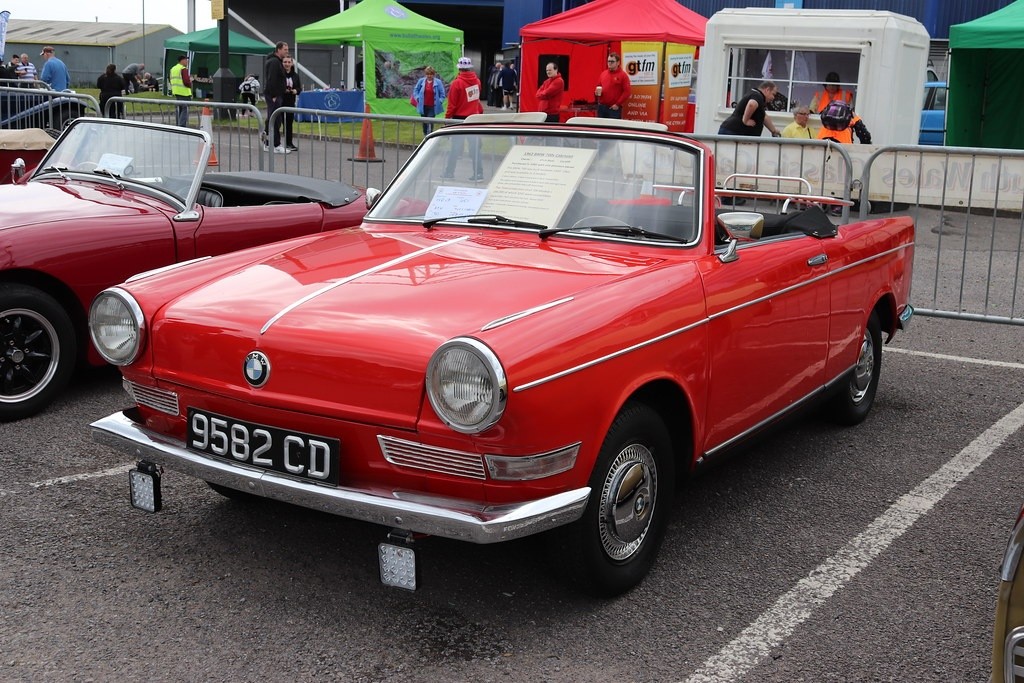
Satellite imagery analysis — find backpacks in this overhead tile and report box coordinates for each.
[819,100,854,131]
[243,79,255,91]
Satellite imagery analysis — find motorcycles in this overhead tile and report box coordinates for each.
[85,119,920,599]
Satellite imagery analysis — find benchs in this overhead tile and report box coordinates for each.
[607,206,788,244]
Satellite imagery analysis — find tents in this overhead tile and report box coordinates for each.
[944,0,1024,150]
[295,0,464,123]
[519,0,709,134]
[163,27,276,97]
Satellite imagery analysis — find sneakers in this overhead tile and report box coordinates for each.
[264,145,269,151]
[287,144,298,150]
[274,145,291,154]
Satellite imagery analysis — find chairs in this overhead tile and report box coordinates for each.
[178,186,223,209]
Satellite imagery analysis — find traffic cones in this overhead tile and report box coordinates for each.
[347,103,386,162]
[190,106,220,166]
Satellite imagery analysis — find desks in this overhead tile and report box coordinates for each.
[294,91,364,122]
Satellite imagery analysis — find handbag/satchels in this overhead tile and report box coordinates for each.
[410,96,417,106]
[487,89,493,105]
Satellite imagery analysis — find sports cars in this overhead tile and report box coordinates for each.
[1,115,432,425]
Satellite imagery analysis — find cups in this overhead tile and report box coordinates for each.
[596,87,602,96]
[311,83,314,91]
[301,84,304,91]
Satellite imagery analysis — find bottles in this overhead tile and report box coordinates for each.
[340,80,345,91]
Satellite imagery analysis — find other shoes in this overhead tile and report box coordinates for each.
[440,173,456,180]
[467,173,484,182]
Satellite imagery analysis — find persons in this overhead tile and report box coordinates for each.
[239,74,260,118]
[97,64,125,120]
[536,61,564,146]
[263,41,291,153]
[487,62,518,110]
[413,66,445,137]
[817,111,872,144]
[282,55,302,151]
[809,72,854,114]
[40,45,70,93]
[781,106,817,139]
[0,52,40,89]
[594,52,630,159]
[437,57,485,183]
[122,63,160,96]
[355,50,402,99]
[717,81,780,137]
[169,54,193,127]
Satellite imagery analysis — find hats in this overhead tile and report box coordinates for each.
[13,55,19,58]
[177,54,190,60]
[457,57,474,69]
[826,72,841,82]
[40,46,55,55]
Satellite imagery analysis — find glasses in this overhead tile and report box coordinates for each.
[609,61,617,62]
[797,112,810,116]
